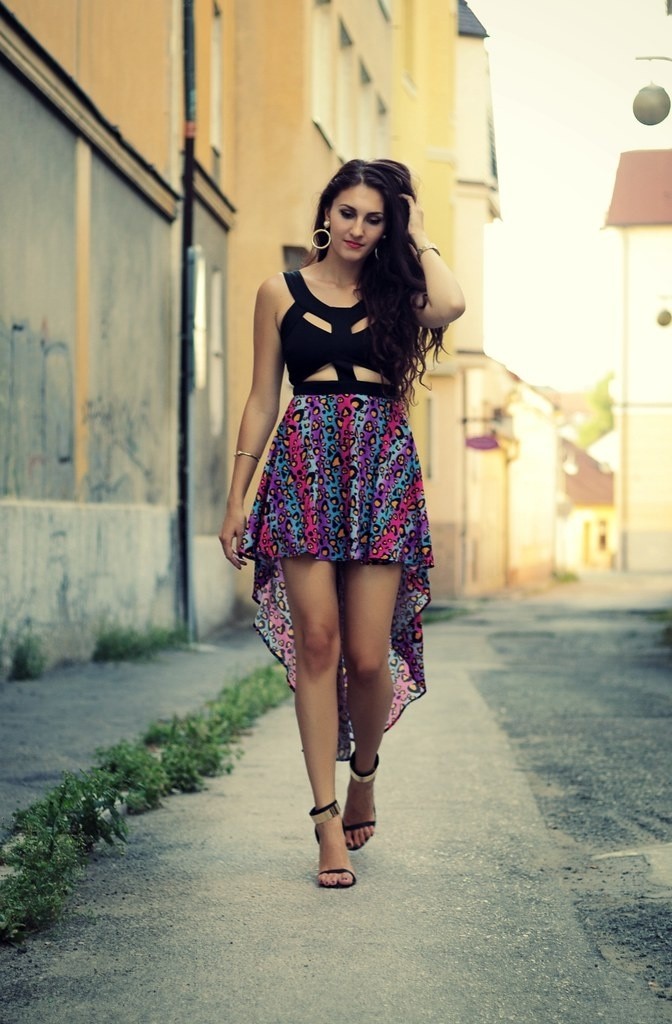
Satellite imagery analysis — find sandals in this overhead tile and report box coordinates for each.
[310,801,356,888]
[343,750,379,851]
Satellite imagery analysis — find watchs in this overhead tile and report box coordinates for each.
[417,242,441,262]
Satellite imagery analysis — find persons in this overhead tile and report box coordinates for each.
[218,160,465,888]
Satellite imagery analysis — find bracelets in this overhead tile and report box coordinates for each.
[233,450,260,462]
